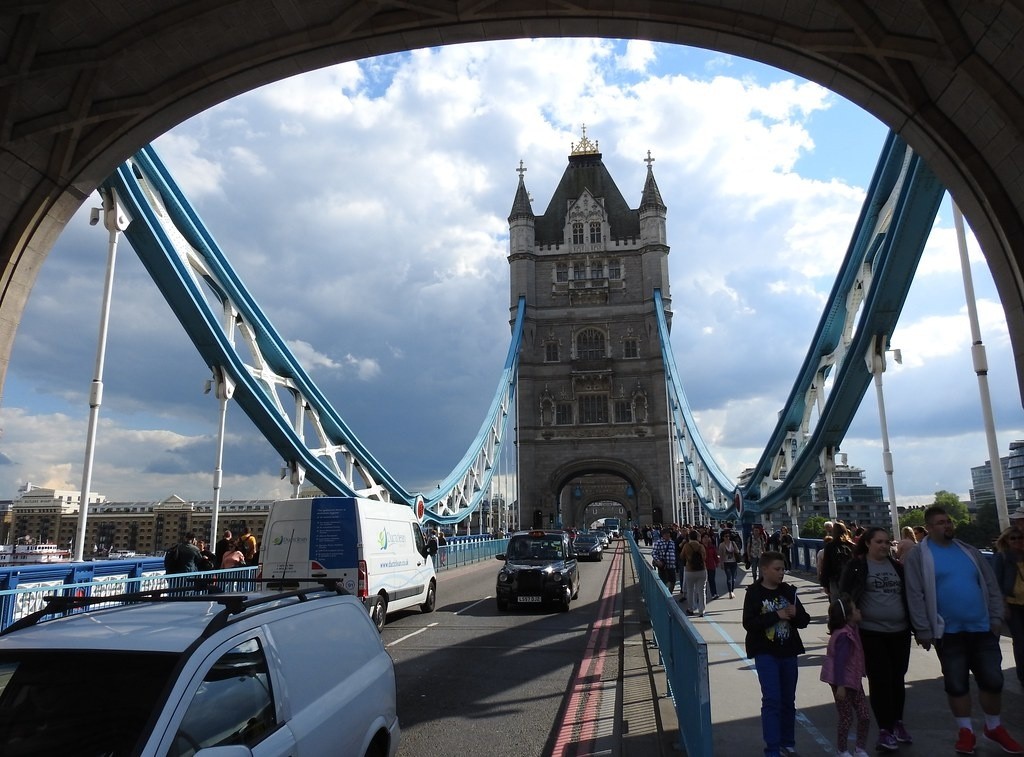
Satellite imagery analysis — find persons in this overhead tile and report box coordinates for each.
[491,525,533,553]
[718,531,740,599]
[680,531,708,617]
[423,530,447,569]
[913,526,928,542]
[839,527,916,749]
[652,529,676,594]
[820,597,871,757]
[771,530,781,551]
[632,520,770,583]
[164,526,261,597]
[904,507,1024,754]
[779,526,794,575]
[817,520,867,603]
[897,526,918,561]
[742,552,811,757]
[992,526,1024,688]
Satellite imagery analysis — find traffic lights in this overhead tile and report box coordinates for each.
[550,513,555,523]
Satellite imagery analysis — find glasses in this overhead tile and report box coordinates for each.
[229,546,234,547]
[722,535,730,537]
[926,520,952,527]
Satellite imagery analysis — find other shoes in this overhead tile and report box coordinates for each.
[779,745,800,757]
[712,594,719,599]
[700,613,704,617]
[679,596,687,602]
[729,593,736,599]
[686,608,694,616]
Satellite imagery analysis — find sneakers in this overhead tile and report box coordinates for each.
[982,723,1024,754]
[893,722,913,742]
[878,727,896,750]
[955,727,977,754]
[836,747,869,757]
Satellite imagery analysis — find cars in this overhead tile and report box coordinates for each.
[589,530,609,549]
[572,534,604,562]
[562,527,580,541]
[496,531,580,612]
[596,524,620,544]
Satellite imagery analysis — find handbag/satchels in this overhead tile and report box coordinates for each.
[197,559,214,570]
[652,556,668,570]
[170,548,195,567]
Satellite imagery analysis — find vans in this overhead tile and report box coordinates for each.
[255,497,439,634]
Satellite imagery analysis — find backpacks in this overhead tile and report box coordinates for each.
[732,533,742,550]
[832,555,849,582]
[686,543,704,570]
[236,535,253,558]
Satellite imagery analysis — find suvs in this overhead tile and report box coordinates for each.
[0,576,400,757]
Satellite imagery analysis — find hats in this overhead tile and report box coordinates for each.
[1006,507,1024,520]
[690,531,697,540]
[223,530,232,537]
[243,526,252,533]
[186,531,195,538]
